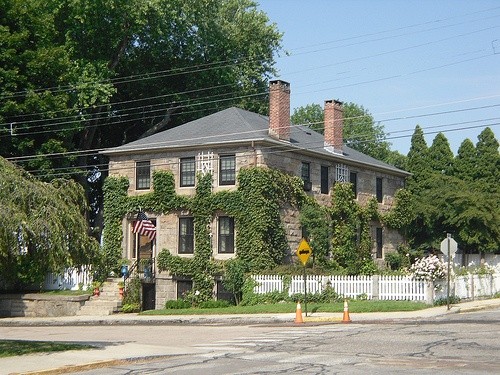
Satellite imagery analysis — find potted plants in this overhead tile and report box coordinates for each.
[141,259,152,277]
[117,257,131,277]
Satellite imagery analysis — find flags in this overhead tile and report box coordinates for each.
[132,211,156,242]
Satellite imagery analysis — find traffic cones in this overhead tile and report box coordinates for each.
[340,299,352,322]
[293,301,304,324]
